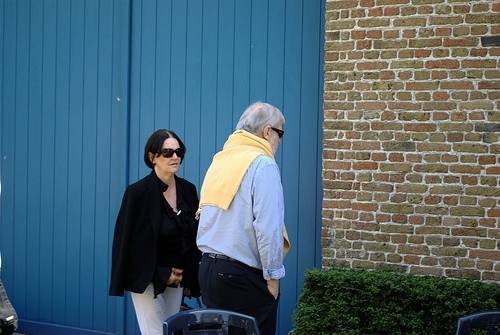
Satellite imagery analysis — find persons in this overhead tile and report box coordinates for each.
[107,129,200,335]
[195,102,286,335]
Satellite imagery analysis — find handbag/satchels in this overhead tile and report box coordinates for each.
[181,292,206,312]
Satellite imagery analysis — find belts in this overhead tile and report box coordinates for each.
[209,253,238,262]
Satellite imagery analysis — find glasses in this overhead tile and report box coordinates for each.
[271,127,285,138]
[159,148,185,158]
